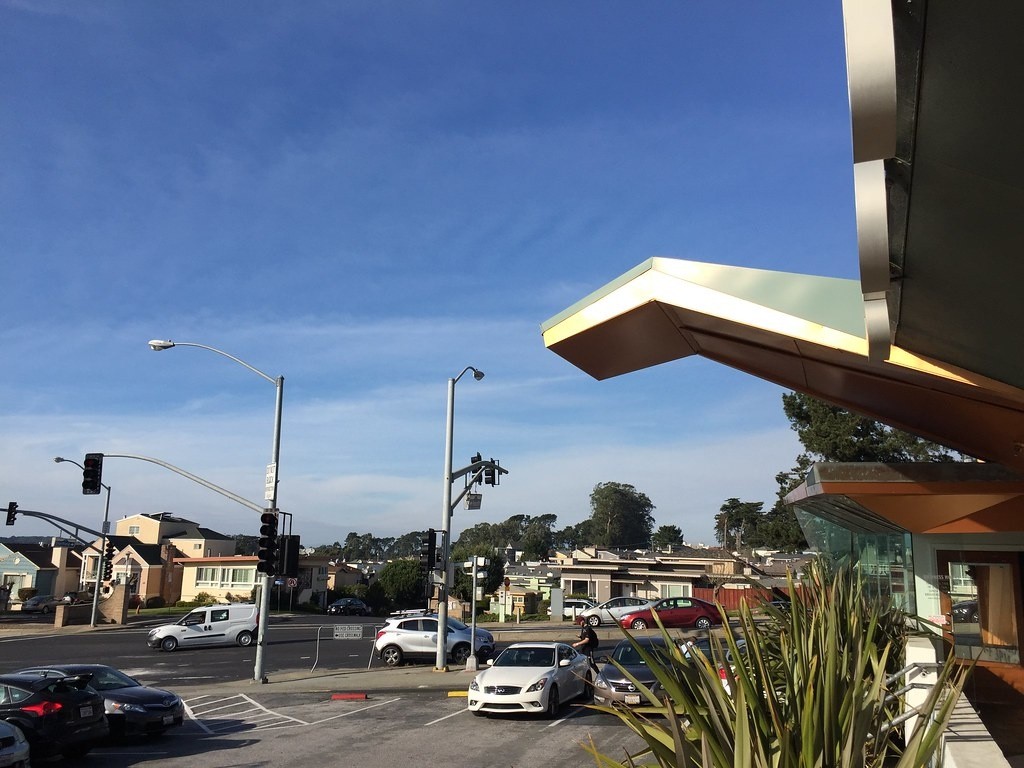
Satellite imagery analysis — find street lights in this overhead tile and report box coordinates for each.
[148,339,285,686]
[53,457,112,627]
[433,366,485,673]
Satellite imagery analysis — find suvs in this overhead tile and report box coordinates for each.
[373,609,496,667]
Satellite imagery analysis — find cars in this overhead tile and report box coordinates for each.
[468,642,593,718]
[0,664,184,768]
[617,597,729,629]
[547,598,611,621]
[327,598,372,617]
[21,594,72,614]
[952,601,979,623]
[580,597,659,627]
[593,637,788,714]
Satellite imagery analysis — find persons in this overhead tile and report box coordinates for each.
[679,636,700,658]
[663,601,674,610]
[199,613,205,624]
[571,615,600,674]
[61,595,72,603]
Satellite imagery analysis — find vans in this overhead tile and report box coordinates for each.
[145,603,260,653]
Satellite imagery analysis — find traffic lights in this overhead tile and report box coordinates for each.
[471,452,482,486]
[485,458,495,487]
[256,508,278,576]
[420,528,436,572]
[103,562,113,581]
[6,502,19,525]
[82,453,104,495]
[105,541,116,561]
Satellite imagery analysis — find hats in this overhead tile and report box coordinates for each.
[576,617,584,625]
